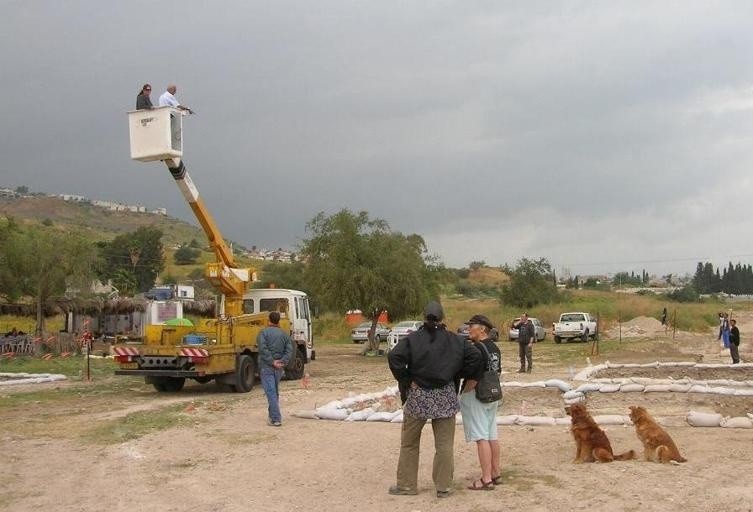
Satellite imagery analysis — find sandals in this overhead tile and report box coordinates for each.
[467,476,503,491]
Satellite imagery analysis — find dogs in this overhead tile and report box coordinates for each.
[627,405,687,463]
[564,402,634,464]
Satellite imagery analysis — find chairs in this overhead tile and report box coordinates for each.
[0,334,33,353]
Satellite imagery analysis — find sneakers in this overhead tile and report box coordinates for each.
[266,419,282,427]
[390,484,417,497]
[436,488,453,498]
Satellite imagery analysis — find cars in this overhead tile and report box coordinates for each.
[342,312,602,350]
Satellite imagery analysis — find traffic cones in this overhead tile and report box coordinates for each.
[301,368,312,392]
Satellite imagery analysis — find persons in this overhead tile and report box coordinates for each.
[385,302,481,498]
[11,328,17,351]
[512,313,534,374]
[718,313,740,364]
[257,312,293,427]
[460,315,502,490]
[159,85,192,147]
[136,85,155,109]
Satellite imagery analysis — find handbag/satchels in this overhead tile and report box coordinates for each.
[474,369,503,404]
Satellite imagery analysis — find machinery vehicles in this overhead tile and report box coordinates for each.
[104,104,315,394]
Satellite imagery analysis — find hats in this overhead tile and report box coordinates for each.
[424,302,445,322]
[268,312,280,324]
[464,314,494,327]
